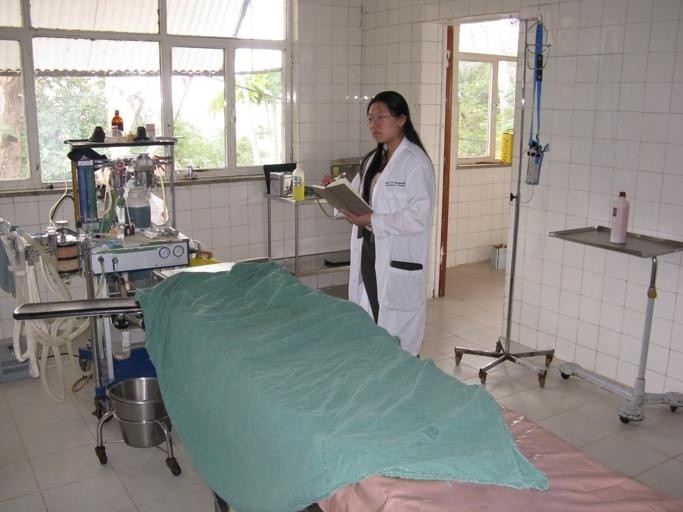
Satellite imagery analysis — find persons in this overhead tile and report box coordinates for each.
[322,92,435,357]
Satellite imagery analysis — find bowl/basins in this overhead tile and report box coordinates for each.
[108,378,167,422]
[111,412,172,449]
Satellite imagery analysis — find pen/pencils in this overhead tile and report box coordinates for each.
[325,171,346,186]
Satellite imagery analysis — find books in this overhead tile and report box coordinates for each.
[309,178,372,217]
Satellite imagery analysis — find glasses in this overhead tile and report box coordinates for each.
[367,113,392,122]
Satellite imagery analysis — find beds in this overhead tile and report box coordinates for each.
[136,259,683,512]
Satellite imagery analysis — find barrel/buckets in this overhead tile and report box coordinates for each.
[105,377,173,449]
